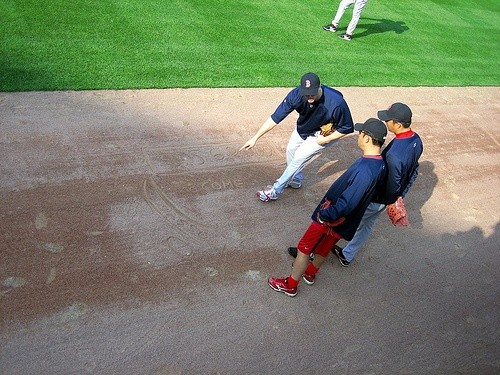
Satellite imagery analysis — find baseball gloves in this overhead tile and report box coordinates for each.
[386,196,410,228]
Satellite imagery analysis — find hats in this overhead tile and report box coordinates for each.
[378,102,412,123]
[355,118,387,141]
[300,72,320,95]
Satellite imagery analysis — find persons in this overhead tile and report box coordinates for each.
[239,73,354,202]
[322,0,368,41]
[267,118,387,297]
[288,102,423,267]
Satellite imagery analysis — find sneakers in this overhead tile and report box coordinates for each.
[323,23,337,32]
[338,33,352,40]
[288,246,315,261]
[292,262,315,284]
[274,178,302,188]
[268,276,297,296]
[332,245,350,266]
[256,189,278,202]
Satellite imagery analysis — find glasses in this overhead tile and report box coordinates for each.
[359,130,374,138]
